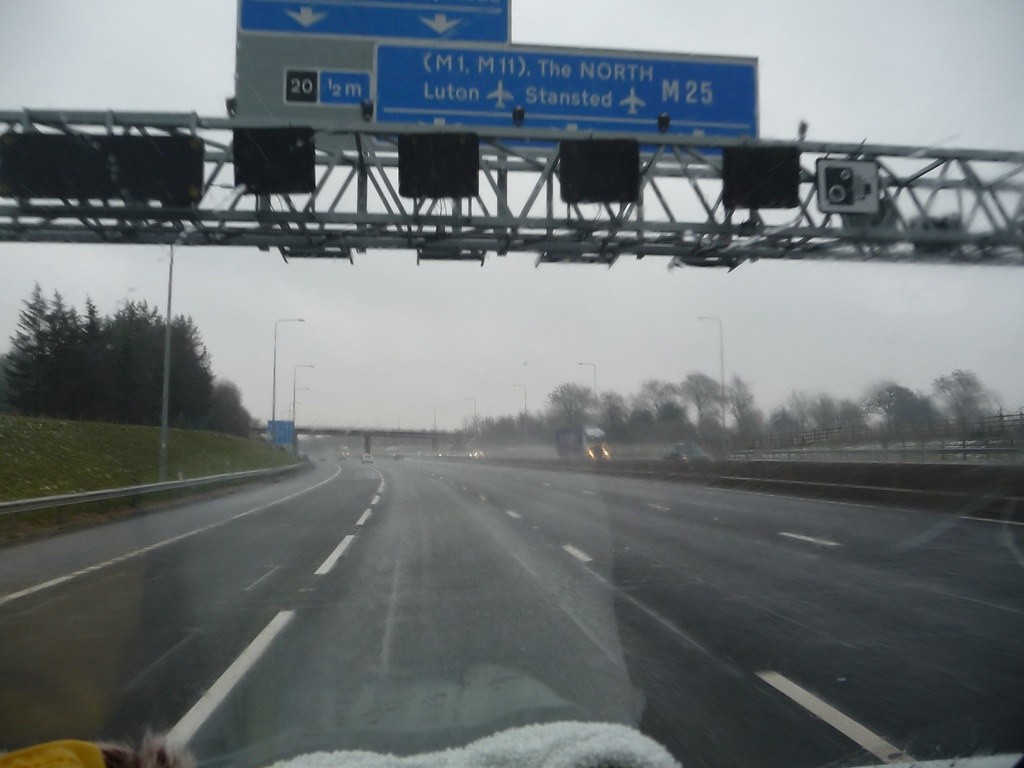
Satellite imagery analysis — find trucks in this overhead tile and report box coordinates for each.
[556,426,608,462]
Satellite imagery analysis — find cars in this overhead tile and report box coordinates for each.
[362,454,373,463]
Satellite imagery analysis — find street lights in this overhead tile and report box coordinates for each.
[577,363,596,399]
[158,183,239,475]
[270,318,305,444]
[513,384,528,446]
[292,364,314,423]
[465,398,479,450]
